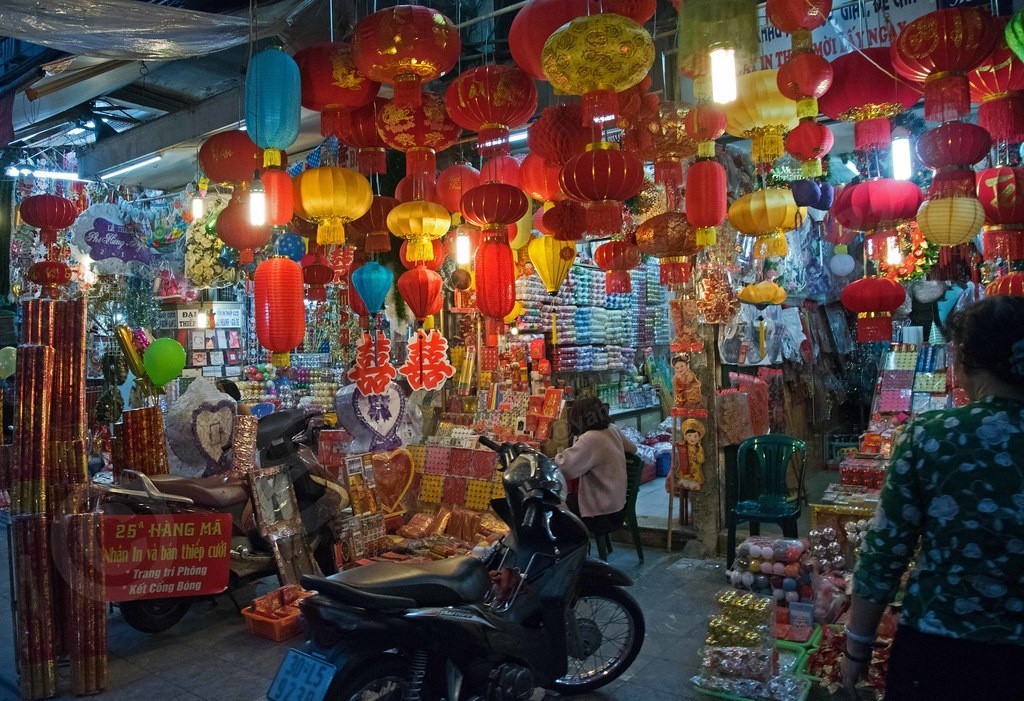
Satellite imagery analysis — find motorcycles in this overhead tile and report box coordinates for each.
[267,434,646,700]
[89,387,353,634]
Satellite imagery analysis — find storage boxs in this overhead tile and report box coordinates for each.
[246,464,302,540]
[241,590,313,643]
[270,526,319,587]
[689,625,840,701]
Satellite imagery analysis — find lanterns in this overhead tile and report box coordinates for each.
[196,0,1024,369]
[20,194,77,243]
[29,247,71,299]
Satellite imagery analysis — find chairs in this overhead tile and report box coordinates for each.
[726,435,809,576]
[590,451,645,567]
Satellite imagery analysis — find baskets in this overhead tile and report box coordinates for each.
[693,618,889,701]
[241,591,314,641]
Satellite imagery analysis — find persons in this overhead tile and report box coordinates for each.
[554,394,637,535]
[842,294,1024,701]
[213,380,241,401]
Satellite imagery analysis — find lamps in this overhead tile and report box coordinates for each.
[101,149,166,179]
[828,244,856,278]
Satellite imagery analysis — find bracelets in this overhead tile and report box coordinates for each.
[841,649,873,665]
[843,623,879,643]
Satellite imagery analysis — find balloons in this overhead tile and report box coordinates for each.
[143,338,186,385]
[274,232,306,263]
